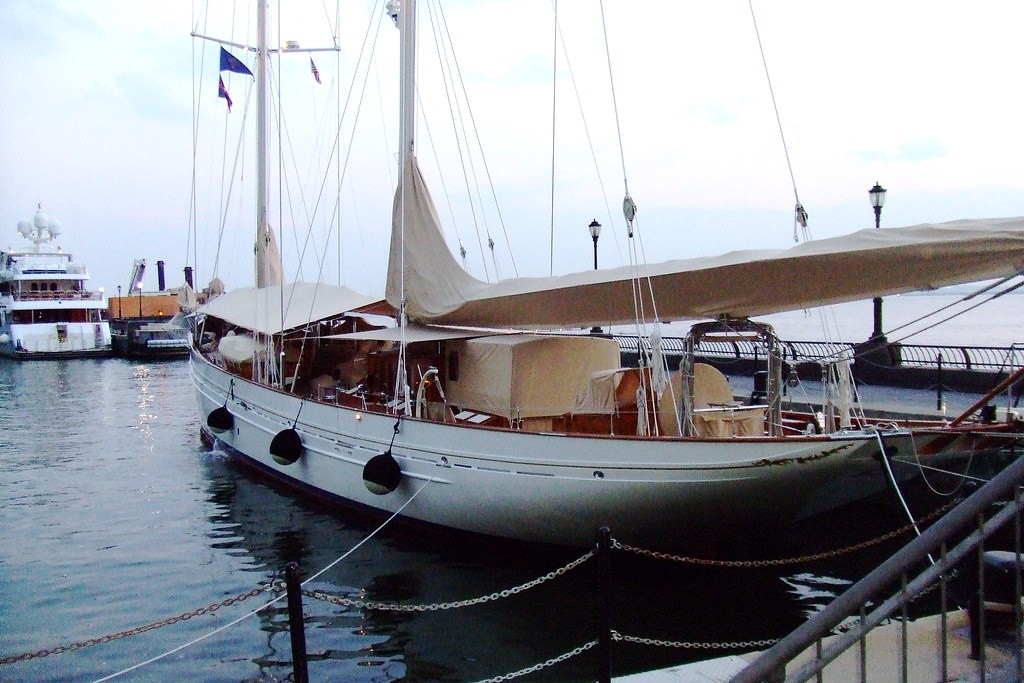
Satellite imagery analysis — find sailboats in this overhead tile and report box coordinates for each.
[181,1,1023,558]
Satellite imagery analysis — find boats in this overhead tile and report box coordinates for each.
[0,203,113,357]
[105,259,200,358]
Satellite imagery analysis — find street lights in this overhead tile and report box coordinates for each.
[866,180,890,343]
[589,219,604,334]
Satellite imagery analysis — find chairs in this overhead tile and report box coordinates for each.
[0,290,94,302]
